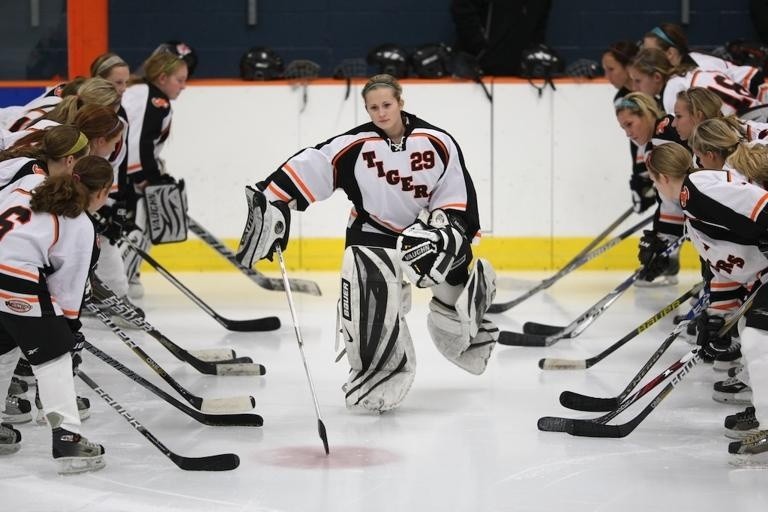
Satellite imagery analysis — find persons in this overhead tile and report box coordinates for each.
[0,156,114,474]
[0,50,192,424]
[645,142,768,468]
[601,17,768,403]
[232,73,500,413]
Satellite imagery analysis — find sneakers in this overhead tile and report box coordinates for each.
[50,426,107,461]
[33,378,90,410]
[81,268,147,322]
[634,254,681,283]
[724,406,762,432]
[713,341,753,395]
[0,356,36,446]
[686,280,743,363]
[727,429,768,456]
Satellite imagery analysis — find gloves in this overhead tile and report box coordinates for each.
[688,309,741,350]
[637,229,670,264]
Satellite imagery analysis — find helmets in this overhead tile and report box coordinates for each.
[240,46,284,82]
[366,43,410,80]
[284,59,323,79]
[412,42,455,78]
[518,43,567,79]
[332,56,380,79]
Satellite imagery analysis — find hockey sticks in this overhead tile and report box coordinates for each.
[559,291,709,412]
[485,213,655,314]
[84,339,264,427]
[83,296,255,414]
[92,275,266,376]
[122,237,281,332]
[276,244,329,455]
[538,281,705,370]
[188,215,321,297]
[73,367,240,471]
[497,232,688,347]
[566,284,762,438]
[537,345,703,432]
[497,206,635,292]
